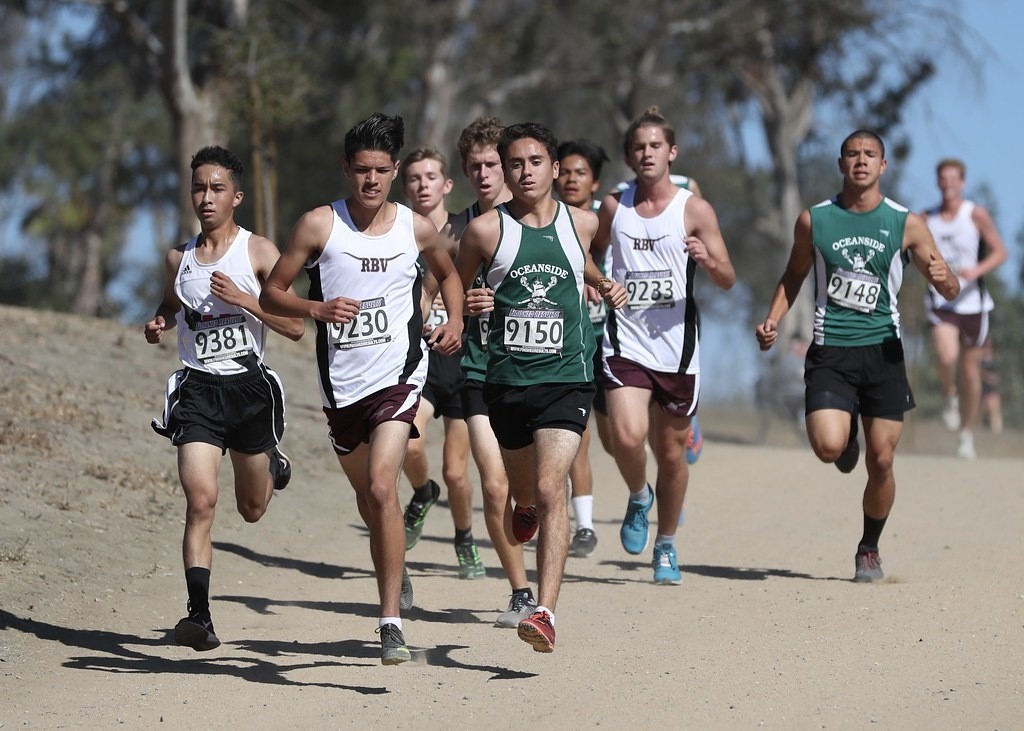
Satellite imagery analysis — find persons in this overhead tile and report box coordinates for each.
[914,161,1006,460]
[978,334,1003,434]
[755,129,962,581]
[144,146,305,652]
[258,111,464,664]
[757,331,809,443]
[399,108,736,651]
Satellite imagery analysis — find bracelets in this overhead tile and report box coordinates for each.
[597,277,612,292]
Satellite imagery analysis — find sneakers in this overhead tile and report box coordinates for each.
[274,446,292,489]
[497,592,537,627]
[517,610,555,653]
[835,412,860,473]
[620,482,654,554]
[572,528,598,557]
[403,479,440,550]
[512,504,538,543]
[400,567,413,611]
[175,611,221,651]
[652,543,683,585]
[454,539,486,580]
[854,551,883,578]
[374,624,411,665]
[686,416,703,464]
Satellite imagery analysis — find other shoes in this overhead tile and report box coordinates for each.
[960,431,976,458]
[942,396,960,431]
